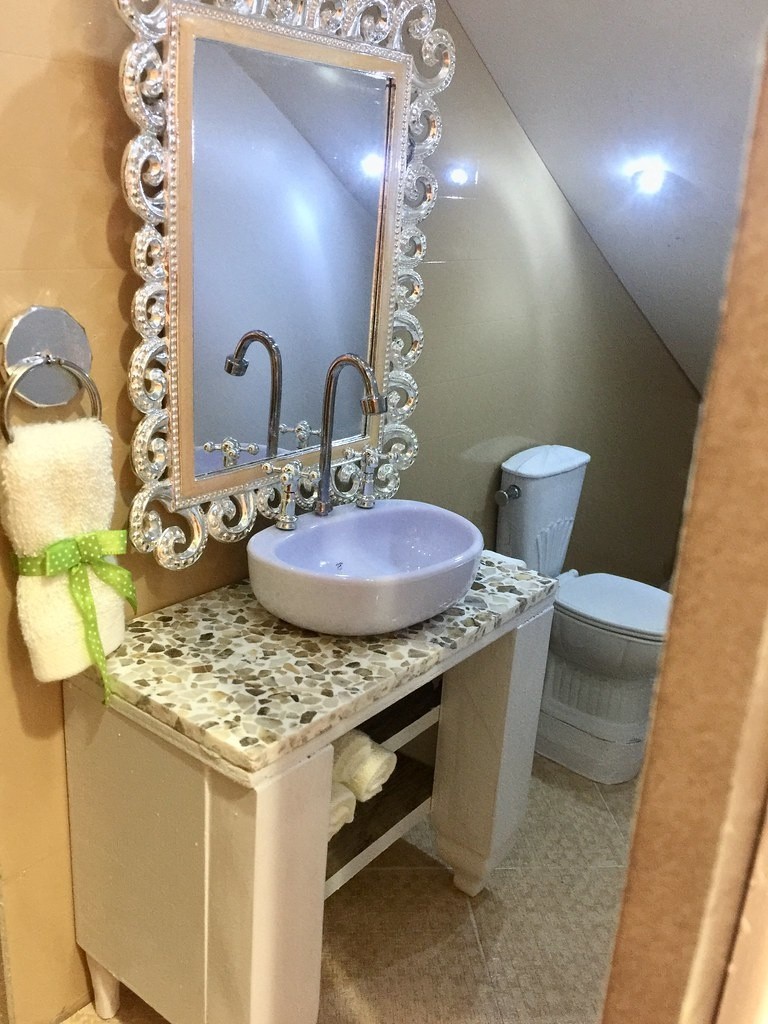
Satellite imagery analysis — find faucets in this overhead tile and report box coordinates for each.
[224,329,282,456]
[313,353,388,517]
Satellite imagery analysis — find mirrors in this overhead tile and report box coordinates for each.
[116,0,457,570]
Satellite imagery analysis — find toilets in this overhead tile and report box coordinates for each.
[495,445,674,786]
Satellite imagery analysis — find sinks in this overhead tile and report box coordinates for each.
[247,498,484,636]
[193,443,292,478]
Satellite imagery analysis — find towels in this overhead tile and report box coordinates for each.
[326,728,398,842]
[0,419,125,681]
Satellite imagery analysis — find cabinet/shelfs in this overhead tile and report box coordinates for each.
[60,550,558,1024]
